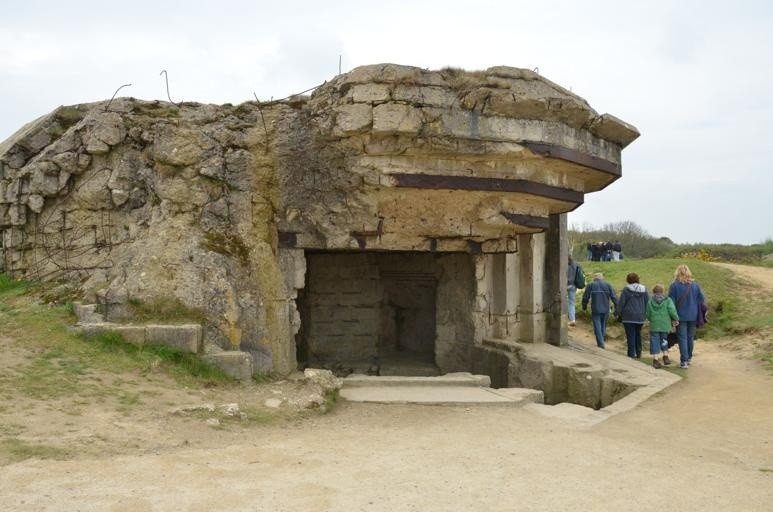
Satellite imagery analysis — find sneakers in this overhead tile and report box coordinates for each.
[680,359,692,369]
[654,356,671,368]
[569,320,576,326]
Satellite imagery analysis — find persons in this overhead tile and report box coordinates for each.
[613,273,648,361]
[646,283,679,368]
[567,250,579,326]
[582,272,618,349]
[666,264,705,369]
[586,238,623,261]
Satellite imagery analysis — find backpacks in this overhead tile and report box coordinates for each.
[576,266,586,289]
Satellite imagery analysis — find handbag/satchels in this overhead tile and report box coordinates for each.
[617,312,623,323]
[668,331,678,348]
